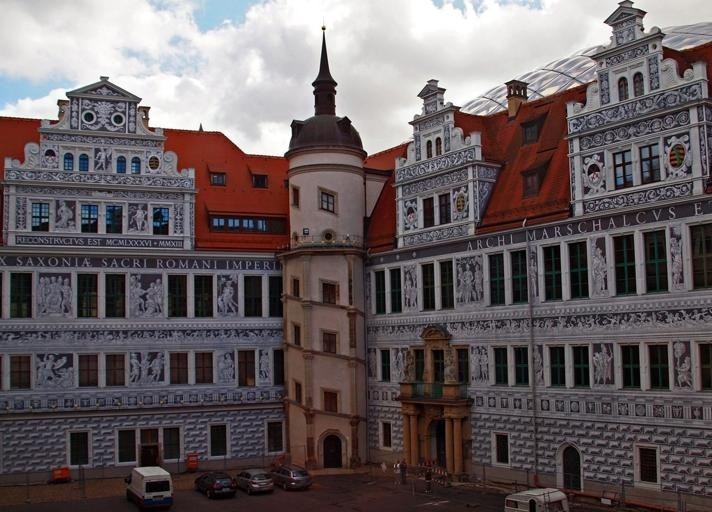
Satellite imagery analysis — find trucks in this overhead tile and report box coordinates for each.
[125,466,175,511]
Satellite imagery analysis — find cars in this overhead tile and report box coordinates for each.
[194,472,236,499]
[270,465,314,490]
[236,468,273,494]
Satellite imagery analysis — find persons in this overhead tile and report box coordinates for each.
[399,458,408,485]
[396,272,417,378]
[393,460,402,488]
[224,353,235,379]
[58,202,72,228]
[529,260,543,386]
[129,353,143,382]
[221,281,237,315]
[35,355,74,386]
[134,205,145,231]
[95,147,108,171]
[259,350,270,378]
[670,237,690,387]
[593,248,614,384]
[36,276,73,313]
[147,353,165,382]
[456,263,489,384]
[129,276,164,316]
